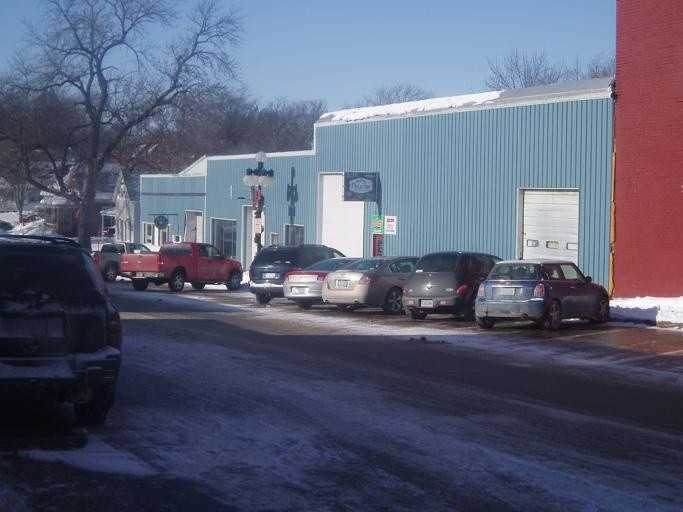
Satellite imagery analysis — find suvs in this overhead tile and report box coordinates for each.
[0,232,122,426]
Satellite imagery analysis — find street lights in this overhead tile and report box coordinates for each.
[243,151,275,254]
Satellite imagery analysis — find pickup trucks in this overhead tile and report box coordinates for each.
[89,242,154,281]
[119,242,243,293]
